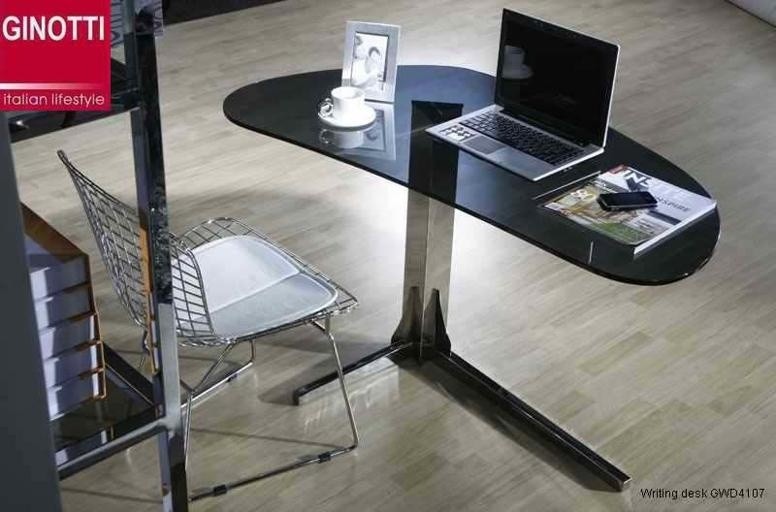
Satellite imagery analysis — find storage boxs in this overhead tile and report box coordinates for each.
[0,0,112,114]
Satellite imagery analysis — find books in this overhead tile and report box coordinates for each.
[534,163,720,257]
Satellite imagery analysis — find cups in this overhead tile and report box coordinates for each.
[320,128,365,150]
[320,86,366,124]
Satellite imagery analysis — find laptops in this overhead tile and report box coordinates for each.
[423,7,621,181]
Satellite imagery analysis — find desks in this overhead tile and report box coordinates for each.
[221,56,721,493]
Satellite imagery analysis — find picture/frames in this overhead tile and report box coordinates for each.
[338,17,404,107]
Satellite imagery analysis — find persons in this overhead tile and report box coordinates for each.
[350,46,383,91]
[350,34,377,90]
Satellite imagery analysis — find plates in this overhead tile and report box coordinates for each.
[317,106,377,131]
[318,121,377,132]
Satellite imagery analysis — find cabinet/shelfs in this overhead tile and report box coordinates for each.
[0,0,195,512]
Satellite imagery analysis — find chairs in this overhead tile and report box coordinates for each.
[51,131,368,504]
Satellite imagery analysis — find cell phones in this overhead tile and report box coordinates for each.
[598,192,656,211]
